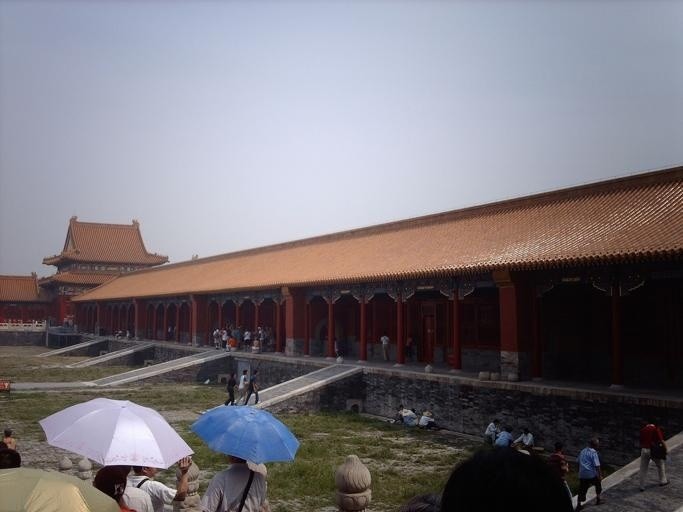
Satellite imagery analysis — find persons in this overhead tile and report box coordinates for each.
[381,333,390,361]
[396,405,605,511]
[225,369,258,406]
[0,428,21,468]
[335,339,339,357]
[93,455,272,512]
[213,325,265,353]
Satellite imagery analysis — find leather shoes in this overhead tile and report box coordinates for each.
[659,479,670,486]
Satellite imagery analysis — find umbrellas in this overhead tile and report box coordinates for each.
[38,398,194,471]
[189,406,301,465]
[1,467,122,512]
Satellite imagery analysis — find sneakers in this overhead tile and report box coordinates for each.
[597,499,605,505]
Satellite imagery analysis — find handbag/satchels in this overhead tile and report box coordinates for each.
[650,440,669,462]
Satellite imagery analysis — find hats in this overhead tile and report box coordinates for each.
[423,411,432,416]
[95,466,131,501]
